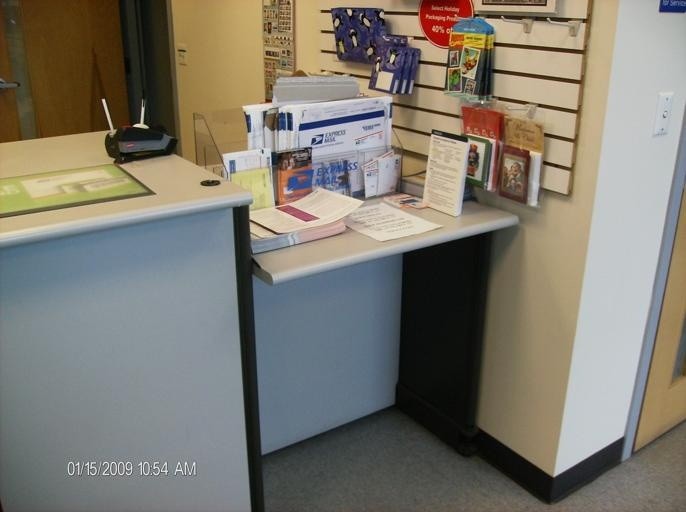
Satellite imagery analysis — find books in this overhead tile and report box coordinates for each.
[497,143,543,208]
[271,145,314,206]
[461,133,495,192]
[421,128,469,216]
[381,191,431,211]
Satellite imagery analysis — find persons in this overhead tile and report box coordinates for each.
[504,161,524,193]
